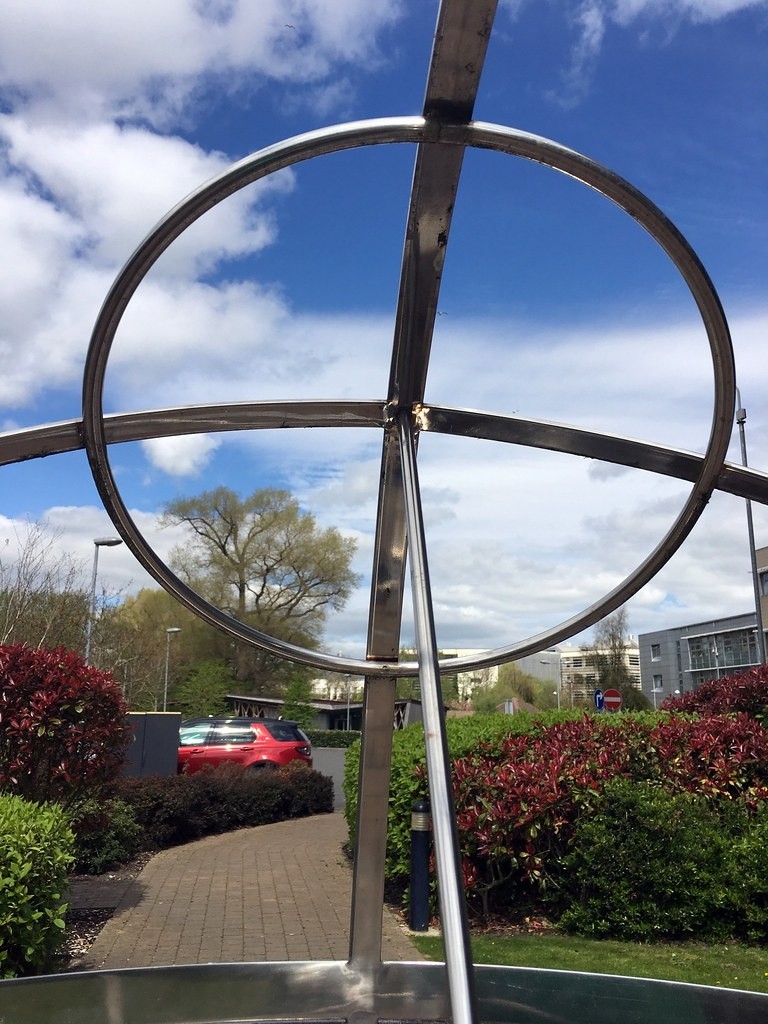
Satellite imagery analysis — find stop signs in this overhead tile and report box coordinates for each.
[603,689,623,711]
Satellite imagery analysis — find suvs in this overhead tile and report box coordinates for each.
[177,715,314,774]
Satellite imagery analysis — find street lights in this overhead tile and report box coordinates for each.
[344,673,352,730]
[84,537,123,666]
[540,660,561,713]
[163,627,182,712]
